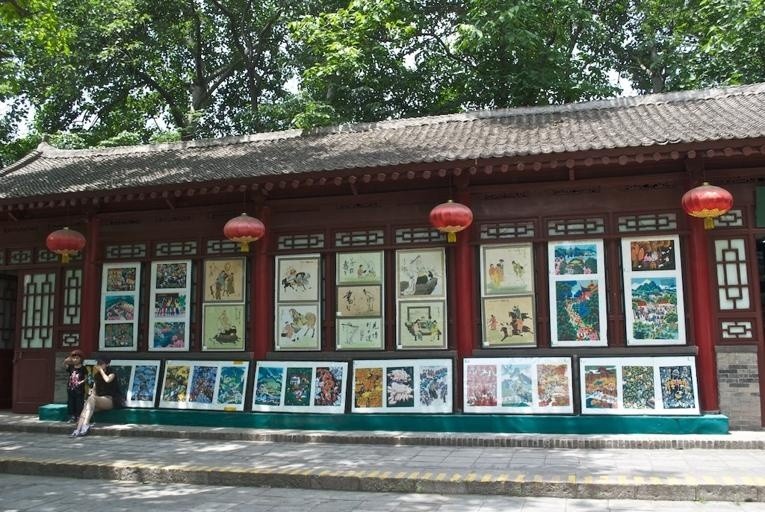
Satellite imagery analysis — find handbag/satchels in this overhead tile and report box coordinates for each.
[113,392,125,409]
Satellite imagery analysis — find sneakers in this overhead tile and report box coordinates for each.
[67,418,90,438]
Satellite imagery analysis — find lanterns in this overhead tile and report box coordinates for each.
[428,200,474,243]
[223,213,266,253]
[46,227,86,263]
[681,181,734,230]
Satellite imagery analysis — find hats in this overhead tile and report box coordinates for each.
[96,354,111,364]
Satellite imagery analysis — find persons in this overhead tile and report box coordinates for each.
[63,350,89,424]
[68,356,118,437]
